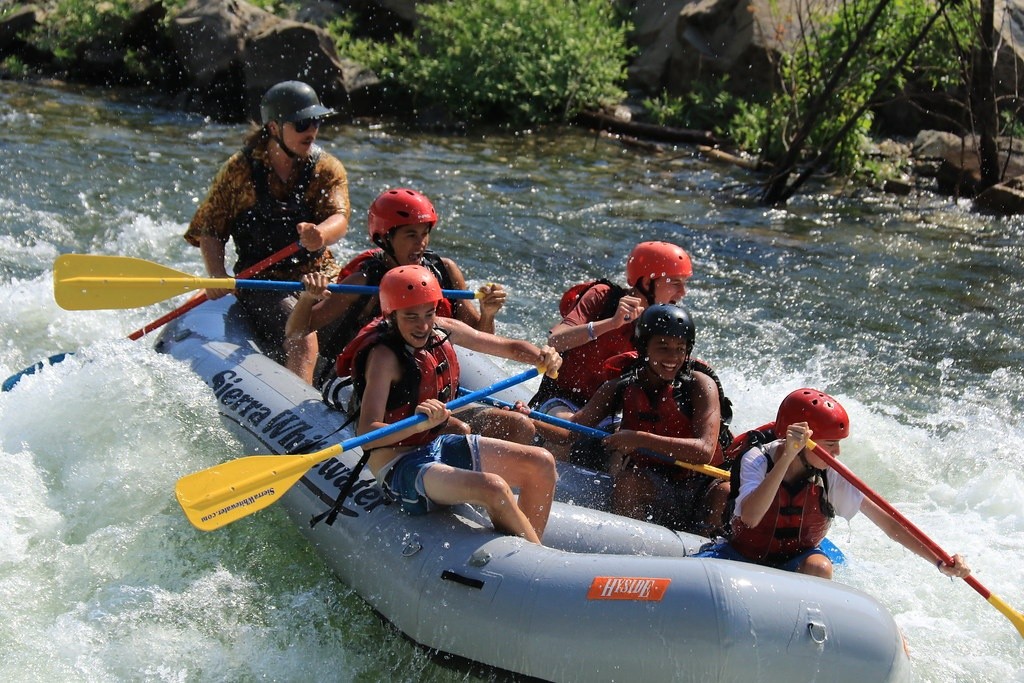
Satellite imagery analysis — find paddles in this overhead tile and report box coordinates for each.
[3,240,302,394]
[805,439,1023,634]
[175,365,543,531]
[54,251,485,312]
[459,387,732,478]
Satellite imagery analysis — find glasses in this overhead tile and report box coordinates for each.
[296,118,321,132]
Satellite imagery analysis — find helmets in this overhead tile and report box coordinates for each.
[774,388,850,441]
[260,80,333,125]
[379,264,444,319]
[626,240,693,286]
[368,188,438,240]
[635,304,695,358]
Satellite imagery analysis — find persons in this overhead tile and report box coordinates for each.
[537,241,693,445]
[185,81,351,385]
[514,304,730,535]
[284,188,506,337]
[699,387,971,584]
[357,265,562,547]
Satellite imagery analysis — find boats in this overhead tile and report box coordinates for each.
[150,272,915,683]
